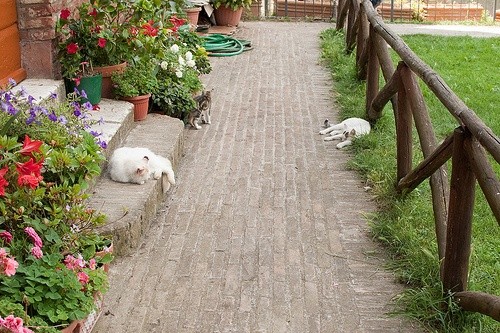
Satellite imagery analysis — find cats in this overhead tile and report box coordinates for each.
[108,147,175,193]
[188,88,214,130]
[319,117,371,150]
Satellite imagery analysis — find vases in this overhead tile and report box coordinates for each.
[186,7,202,32]
[96,238,114,273]
[120,92,151,122]
[86,60,126,99]
[65,71,102,105]
[61,262,107,333]
[59,238,75,256]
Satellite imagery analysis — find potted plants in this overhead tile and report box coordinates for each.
[211,0,261,26]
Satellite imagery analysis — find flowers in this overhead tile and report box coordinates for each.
[0,0,210,333]
